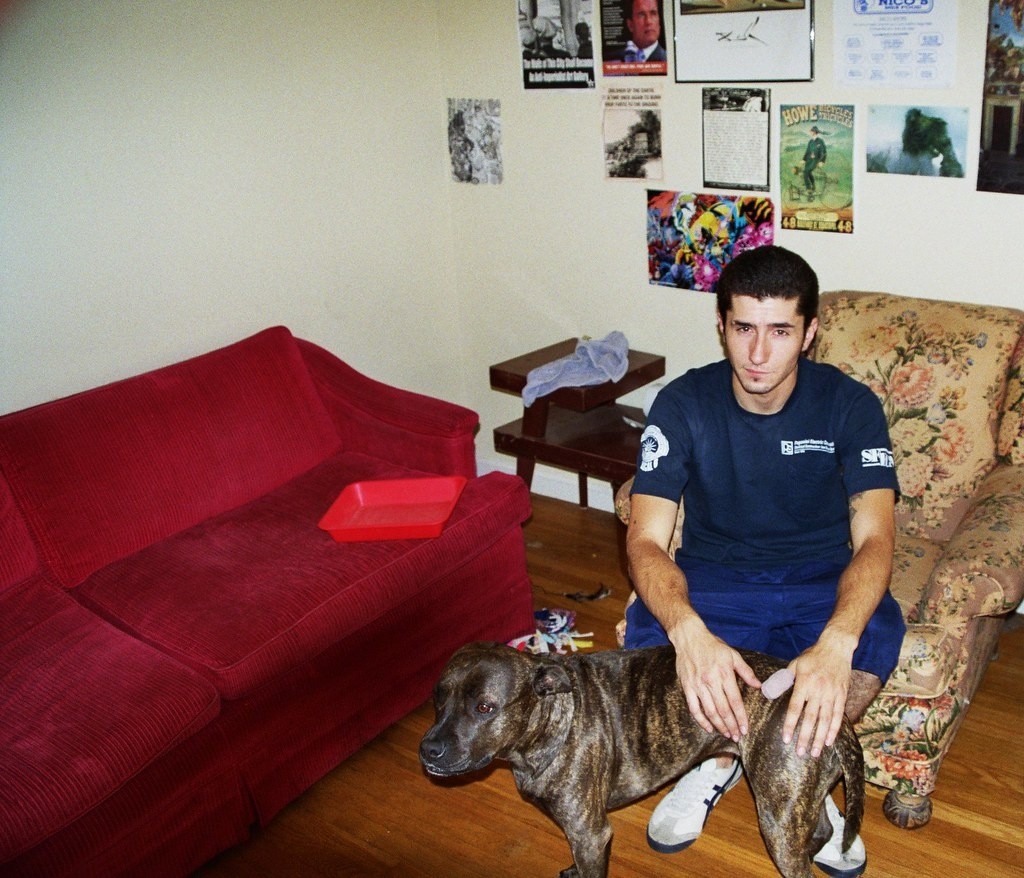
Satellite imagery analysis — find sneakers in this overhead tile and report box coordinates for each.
[810,793,866,878]
[646,754,745,854]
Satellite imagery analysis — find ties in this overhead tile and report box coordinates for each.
[637,50,644,61]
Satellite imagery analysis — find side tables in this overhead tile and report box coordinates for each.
[488,337,666,574]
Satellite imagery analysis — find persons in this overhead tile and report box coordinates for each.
[623,242,907,878]
[574,22,593,59]
[620,0,667,76]
[533,17,572,60]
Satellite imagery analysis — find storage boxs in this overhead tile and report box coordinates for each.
[317,476,467,541]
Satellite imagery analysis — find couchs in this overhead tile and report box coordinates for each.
[612,289,1024,829]
[0,325,537,878]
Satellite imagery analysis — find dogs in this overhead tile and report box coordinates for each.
[417,639,867,878]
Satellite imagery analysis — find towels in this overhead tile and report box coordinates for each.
[521,330,628,408]
